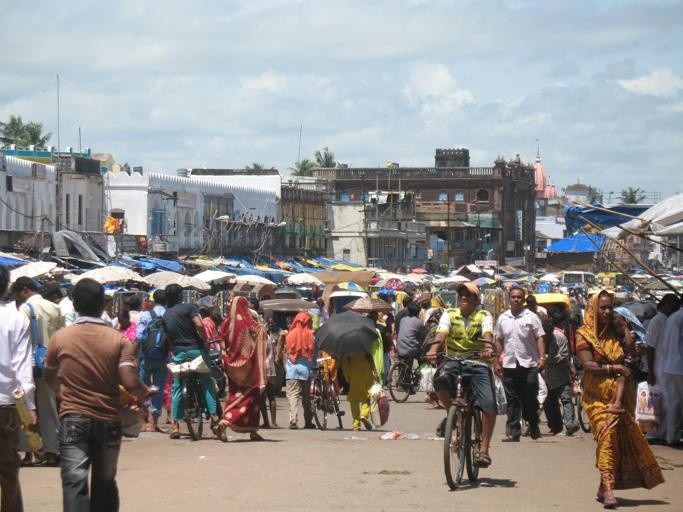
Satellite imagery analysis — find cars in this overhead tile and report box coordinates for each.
[560,265,682,289]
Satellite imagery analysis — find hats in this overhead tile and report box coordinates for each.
[456,282,479,300]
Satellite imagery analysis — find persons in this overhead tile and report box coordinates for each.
[569,281,683,506]
[0,266,159,512]
[135,284,587,467]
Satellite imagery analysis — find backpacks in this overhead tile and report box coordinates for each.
[143,308,167,363]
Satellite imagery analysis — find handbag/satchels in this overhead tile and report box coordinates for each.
[492,375,507,415]
[372,395,389,427]
[32,345,47,373]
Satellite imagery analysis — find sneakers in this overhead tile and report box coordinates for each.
[502,435,521,442]
[567,422,580,435]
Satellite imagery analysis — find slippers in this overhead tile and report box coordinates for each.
[251,436,264,441]
[209,417,228,441]
[171,430,179,439]
[289,424,297,429]
[597,494,617,508]
[361,416,373,431]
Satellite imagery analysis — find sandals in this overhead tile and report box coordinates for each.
[436,414,458,437]
[478,452,491,465]
[142,425,158,433]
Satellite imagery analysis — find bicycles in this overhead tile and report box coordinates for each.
[166,339,223,439]
[387,349,430,403]
[307,353,346,431]
[419,337,498,489]
[509,366,593,437]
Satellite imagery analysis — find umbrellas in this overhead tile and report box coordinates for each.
[543,232,606,265]
